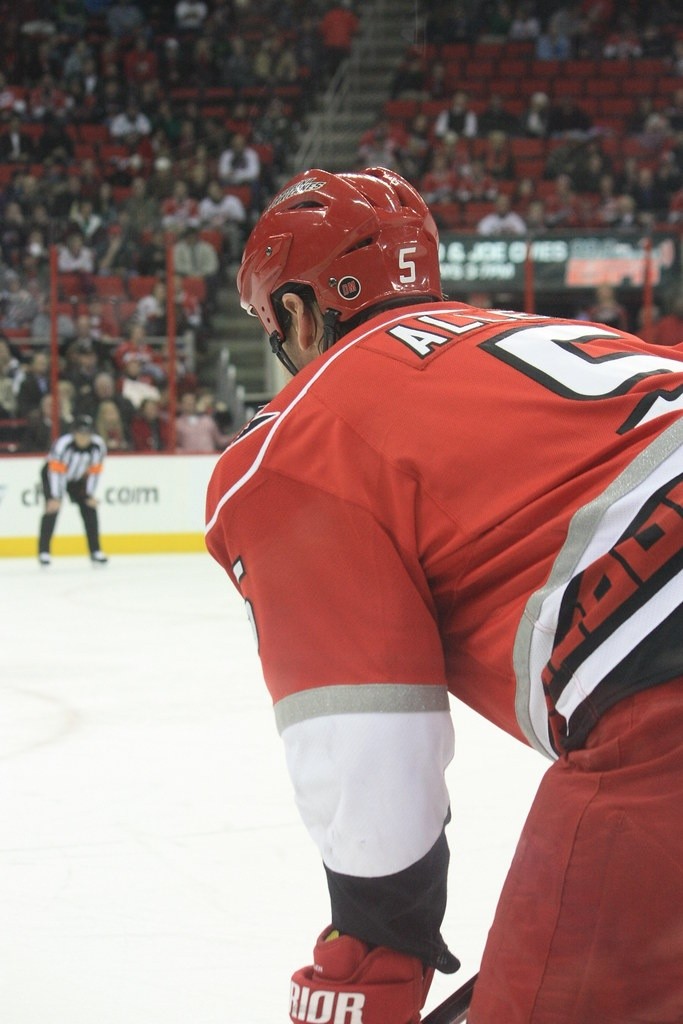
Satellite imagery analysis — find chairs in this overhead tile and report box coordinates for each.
[0,2,681,457]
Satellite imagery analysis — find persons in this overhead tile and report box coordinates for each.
[217,133,261,183]
[172,227,220,312]
[32,293,74,339]
[198,182,246,258]
[542,174,581,227]
[37,415,109,563]
[130,397,177,453]
[158,181,200,229]
[203,166,683,1024]
[532,23,571,62]
[57,230,94,275]
[519,92,549,139]
[432,90,478,137]
[475,91,535,139]
[544,96,596,133]
[0,0,683,455]
[119,174,159,231]
[477,194,527,235]
[172,393,242,451]
[106,101,153,137]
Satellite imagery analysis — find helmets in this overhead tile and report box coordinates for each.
[71,414,94,433]
[236,165,441,347]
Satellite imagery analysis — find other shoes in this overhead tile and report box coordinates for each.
[91,551,107,562]
[40,551,50,563]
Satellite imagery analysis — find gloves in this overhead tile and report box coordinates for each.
[288,925,436,1024]
